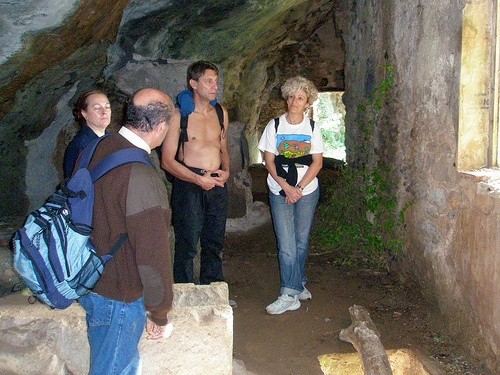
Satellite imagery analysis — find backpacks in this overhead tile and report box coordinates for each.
[12,134,154,309]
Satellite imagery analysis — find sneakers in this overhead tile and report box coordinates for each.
[265,294,301,314]
[298,287,312,301]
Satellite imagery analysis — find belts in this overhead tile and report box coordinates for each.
[192,167,218,177]
